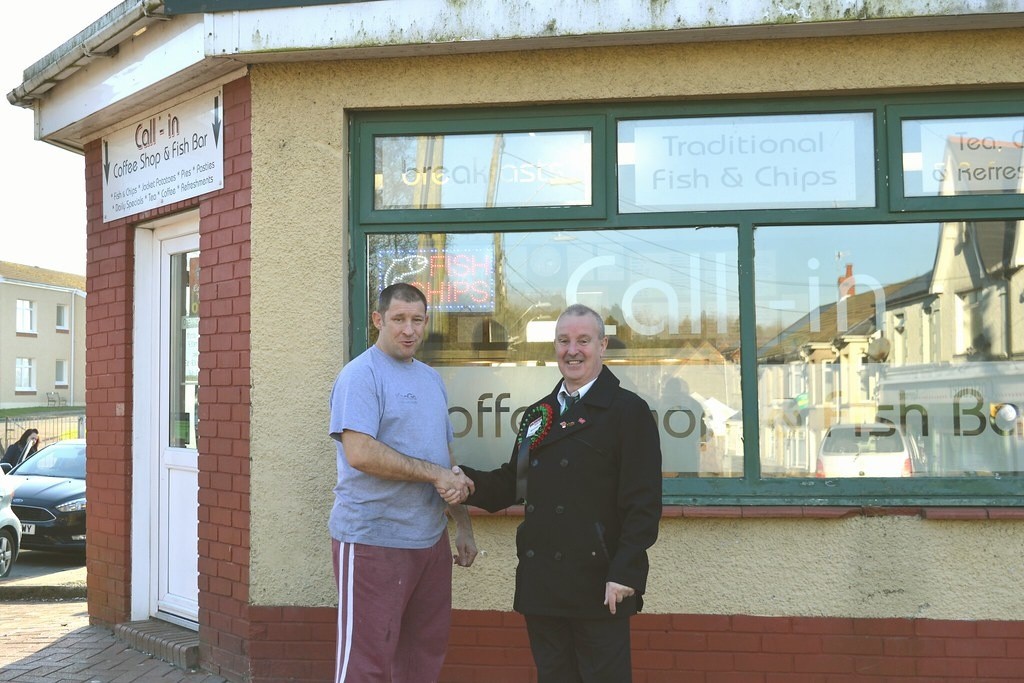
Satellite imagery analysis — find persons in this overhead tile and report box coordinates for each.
[325,282,478,683]
[436,304,663,683]
[1,429,39,474]
[604,338,707,478]
[419,319,509,369]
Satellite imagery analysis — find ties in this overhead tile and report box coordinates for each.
[561,395,579,415]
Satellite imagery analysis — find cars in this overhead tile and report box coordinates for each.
[0,466,23,578]
[1,439,86,566]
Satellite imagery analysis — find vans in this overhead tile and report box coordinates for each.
[818,423,927,478]
